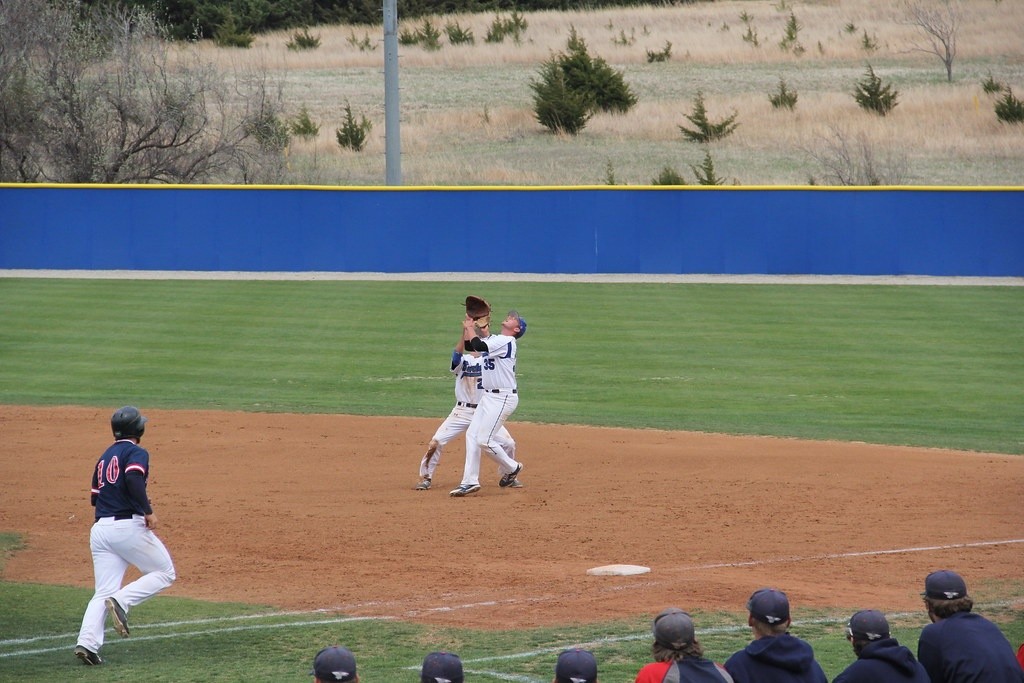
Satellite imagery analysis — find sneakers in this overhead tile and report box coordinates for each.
[105,597,130,638]
[511,478,523,488]
[499,463,523,488]
[73,645,102,665]
[415,478,432,490]
[449,484,481,496]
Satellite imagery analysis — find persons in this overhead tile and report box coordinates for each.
[450,308,527,496]
[723,588,829,683]
[917,570,1024,683]
[635,607,734,683]
[832,609,931,683]
[415,312,523,490]
[74,406,177,666]
[553,648,599,683]
[313,646,464,683]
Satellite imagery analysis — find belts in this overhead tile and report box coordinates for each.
[486,389,517,393]
[458,402,478,408]
[95,514,133,522]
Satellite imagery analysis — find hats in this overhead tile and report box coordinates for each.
[651,608,694,649]
[310,646,356,683]
[919,569,967,600]
[419,651,464,683]
[555,648,597,683]
[508,310,527,334]
[846,609,889,640]
[746,588,789,623]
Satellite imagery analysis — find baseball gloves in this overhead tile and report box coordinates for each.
[466,295,492,329]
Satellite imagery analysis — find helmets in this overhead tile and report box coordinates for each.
[111,405,147,441]
